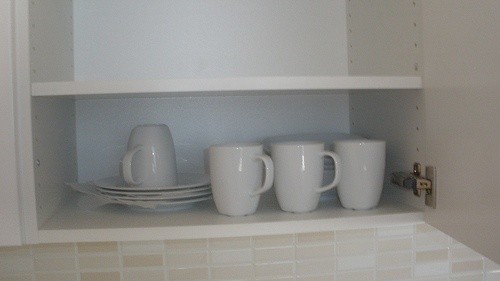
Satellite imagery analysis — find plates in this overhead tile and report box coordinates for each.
[92,173,212,212]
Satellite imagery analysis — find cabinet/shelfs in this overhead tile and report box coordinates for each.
[13,0,500,266]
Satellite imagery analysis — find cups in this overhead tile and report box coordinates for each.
[271,140,341,212]
[119,124,177,187]
[332,139,388,211]
[203,141,274,217]
